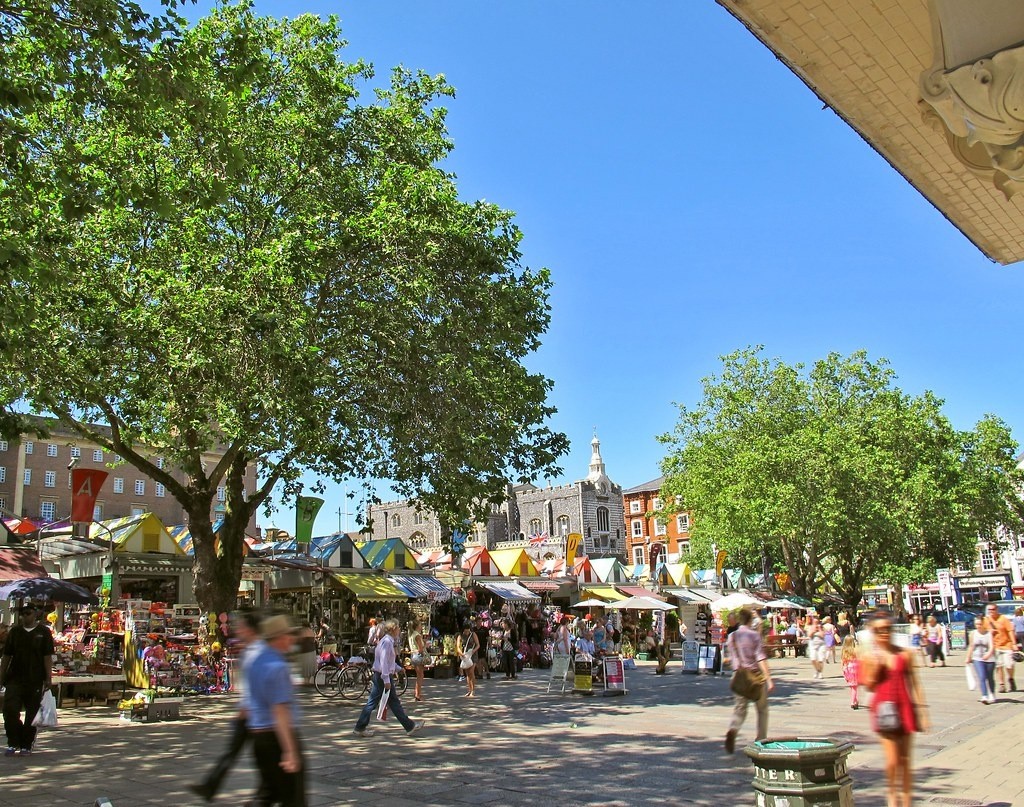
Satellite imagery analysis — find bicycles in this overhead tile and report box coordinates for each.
[313,649,409,700]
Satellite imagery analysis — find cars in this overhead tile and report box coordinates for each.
[859,600,1024,643]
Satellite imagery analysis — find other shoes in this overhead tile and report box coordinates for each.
[813,671,824,679]
[185,784,214,799]
[725,729,735,753]
[352,728,375,736]
[826,659,837,664]
[931,660,946,668]
[977,682,1018,704]
[850,701,858,711]
[459,673,517,697]
[5,745,33,756]
[405,721,424,735]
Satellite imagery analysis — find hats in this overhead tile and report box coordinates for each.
[21,603,36,610]
[258,613,298,639]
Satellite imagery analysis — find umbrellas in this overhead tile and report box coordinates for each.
[570,596,677,611]
[0,576,100,605]
[712,593,806,609]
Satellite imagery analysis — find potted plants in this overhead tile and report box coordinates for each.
[638,637,652,660]
[765,623,797,643]
[622,635,634,659]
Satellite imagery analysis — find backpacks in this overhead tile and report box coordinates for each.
[606,624,621,644]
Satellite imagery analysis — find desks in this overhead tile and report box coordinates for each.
[763,643,808,658]
[51,674,127,708]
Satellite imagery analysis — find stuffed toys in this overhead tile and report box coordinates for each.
[456,592,502,646]
[47,587,110,632]
[144,612,229,692]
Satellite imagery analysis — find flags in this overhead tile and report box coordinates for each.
[70,467,109,526]
[296,496,729,580]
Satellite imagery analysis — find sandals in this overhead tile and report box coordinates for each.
[414,695,424,701]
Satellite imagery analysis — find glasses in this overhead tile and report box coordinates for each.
[21,610,34,616]
[871,626,893,634]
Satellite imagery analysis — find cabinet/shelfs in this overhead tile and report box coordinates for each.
[331,599,363,657]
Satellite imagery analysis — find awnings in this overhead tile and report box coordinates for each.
[0,547,48,582]
[335,574,725,605]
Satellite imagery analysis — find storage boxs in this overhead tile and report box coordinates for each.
[92,698,108,706]
[76,698,92,706]
[60,697,76,708]
[52,598,201,688]
[118,703,180,721]
[107,693,125,700]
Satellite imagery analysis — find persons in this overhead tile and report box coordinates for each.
[457,624,480,696]
[317,617,332,638]
[913,604,1018,705]
[653,617,687,675]
[186,610,263,807]
[239,614,307,807]
[408,620,426,700]
[837,613,931,807]
[367,613,386,646]
[575,615,614,683]
[724,610,837,753]
[557,617,571,653]
[473,617,493,680]
[353,618,425,737]
[500,617,517,680]
[0,602,55,756]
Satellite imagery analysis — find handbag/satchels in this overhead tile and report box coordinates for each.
[878,701,900,733]
[831,624,842,645]
[376,687,389,723]
[460,648,473,669]
[32,690,58,729]
[410,647,432,666]
[502,640,514,652]
[730,667,763,701]
[965,662,977,691]
